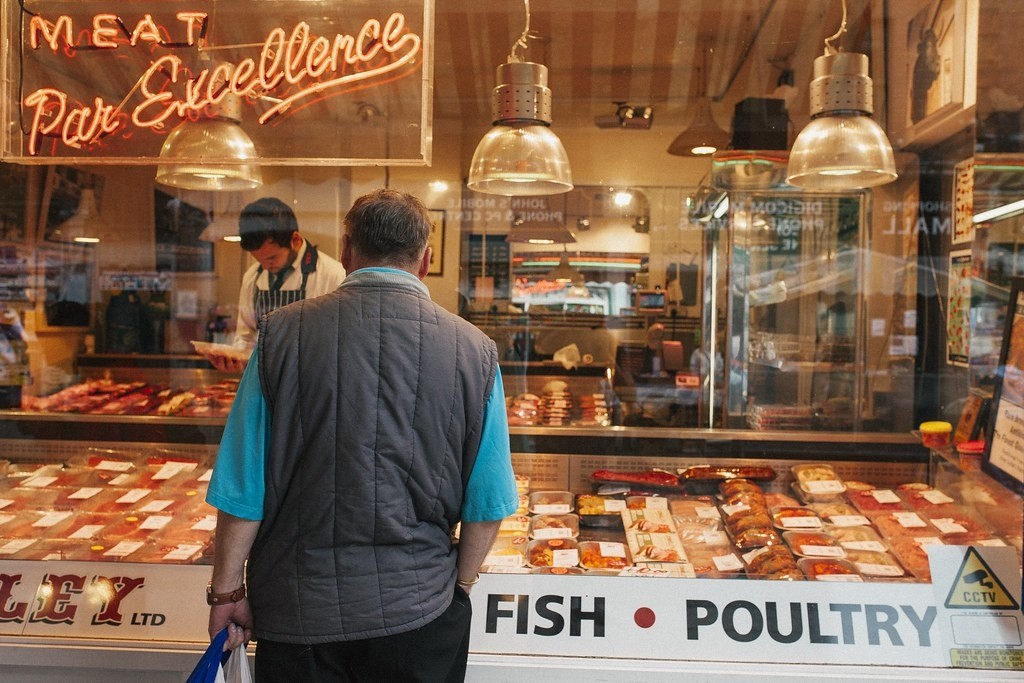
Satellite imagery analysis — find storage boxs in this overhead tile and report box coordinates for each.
[0,446,214,563]
[481,462,1022,583]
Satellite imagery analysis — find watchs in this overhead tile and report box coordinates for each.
[206,579,246,606]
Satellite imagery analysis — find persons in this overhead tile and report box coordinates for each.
[195,194,347,379]
[202,187,520,683]
[689,328,726,428]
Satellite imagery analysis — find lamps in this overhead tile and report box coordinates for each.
[668,101,730,158]
[50,174,124,244]
[466,0,574,197]
[199,191,243,243]
[543,193,584,283]
[785,0,898,192]
[506,198,577,245]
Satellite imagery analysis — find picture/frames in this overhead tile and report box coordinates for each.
[36,250,96,333]
[145,177,221,277]
[35,166,110,251]
[426,208,447,276]
[980,273,1024,498]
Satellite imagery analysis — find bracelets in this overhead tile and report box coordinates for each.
[455,572,480,585]
